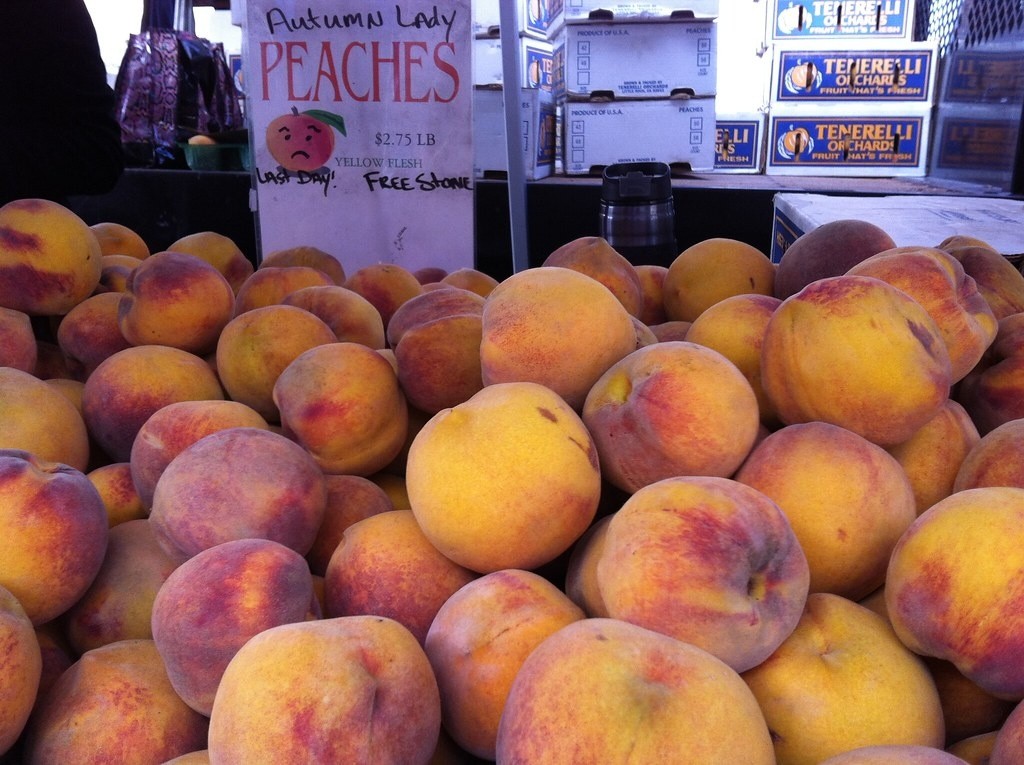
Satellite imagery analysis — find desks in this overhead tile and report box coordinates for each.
[122,168,258,270]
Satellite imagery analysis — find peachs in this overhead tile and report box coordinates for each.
[0,198,1024,765]
[187,135,216,144]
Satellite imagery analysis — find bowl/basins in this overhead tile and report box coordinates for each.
[178,143,245,172]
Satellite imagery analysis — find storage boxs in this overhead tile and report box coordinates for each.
[476,0,1024,266]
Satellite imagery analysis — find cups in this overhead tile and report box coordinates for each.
[600,162,677,268]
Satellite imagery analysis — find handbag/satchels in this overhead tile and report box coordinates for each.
[112,29,244,146]
